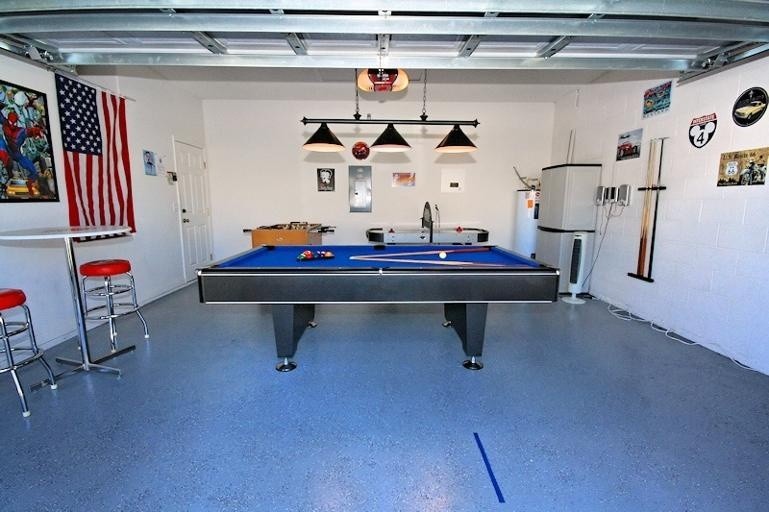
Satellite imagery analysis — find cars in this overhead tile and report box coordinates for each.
[733,100,765,122]
[643,80,672,115]
[618,141,640,158]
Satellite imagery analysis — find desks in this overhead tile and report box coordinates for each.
[0,226,136,392]
[195,244,560,370]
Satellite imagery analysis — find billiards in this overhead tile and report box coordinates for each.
[297,250,334,261]
[439,252,447,258]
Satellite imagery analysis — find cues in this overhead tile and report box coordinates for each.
[352,247,489,257]
[637,138,658,276]
[349,256,505,267]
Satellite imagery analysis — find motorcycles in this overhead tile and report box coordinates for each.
[737,156,767,184]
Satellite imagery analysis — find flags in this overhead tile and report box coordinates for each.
[54,70,135,242]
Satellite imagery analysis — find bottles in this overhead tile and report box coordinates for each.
[456,227,462,232]
[389,228,395,245]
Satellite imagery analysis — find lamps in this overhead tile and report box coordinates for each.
[299,68,480,153]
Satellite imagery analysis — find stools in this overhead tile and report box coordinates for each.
[78,259,150,353]
[0,287,57,417]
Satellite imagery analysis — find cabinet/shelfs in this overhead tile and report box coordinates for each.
[252,224,322,248]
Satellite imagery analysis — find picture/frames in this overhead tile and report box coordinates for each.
[0,78,61,204]
